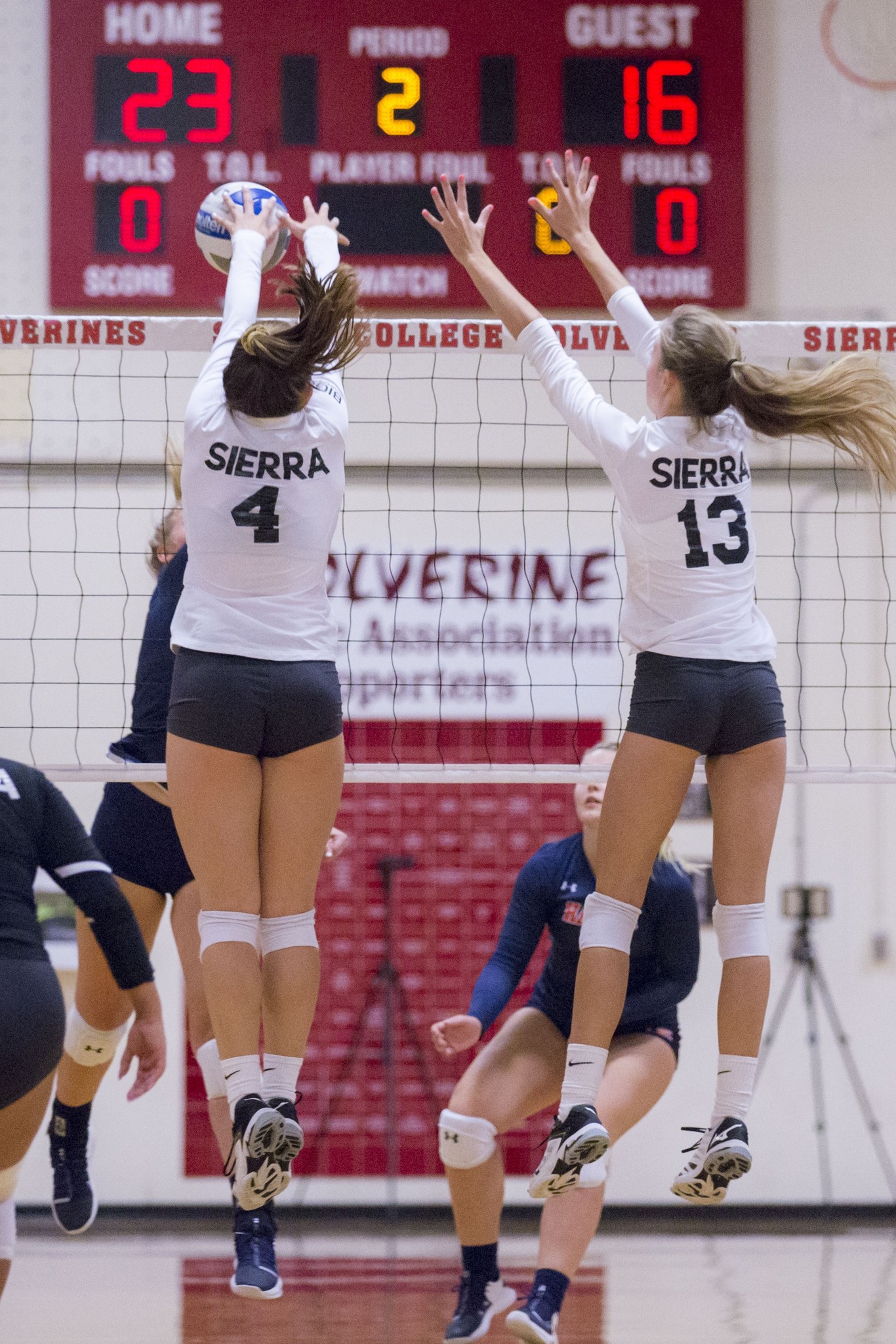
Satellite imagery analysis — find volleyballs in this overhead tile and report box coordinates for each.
[194,181,292,275]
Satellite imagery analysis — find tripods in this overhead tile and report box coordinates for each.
[280,873,443,1322]
[689,923,896,1234]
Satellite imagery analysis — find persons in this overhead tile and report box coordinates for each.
[419,151,890,1203]
[35,462,293,1305]
[169,192,357,1215]
[435,742,704,1343]
[0,749,172,1297]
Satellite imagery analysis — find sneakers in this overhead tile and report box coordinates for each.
[228,1212,284,1300]
[505,1285,559,1344]
[224,1093,305,1212]
[50,1138,99,1235]
[670,1116,753,1206]
[443,1263,518,1344]
[526,1103,609,1197]
